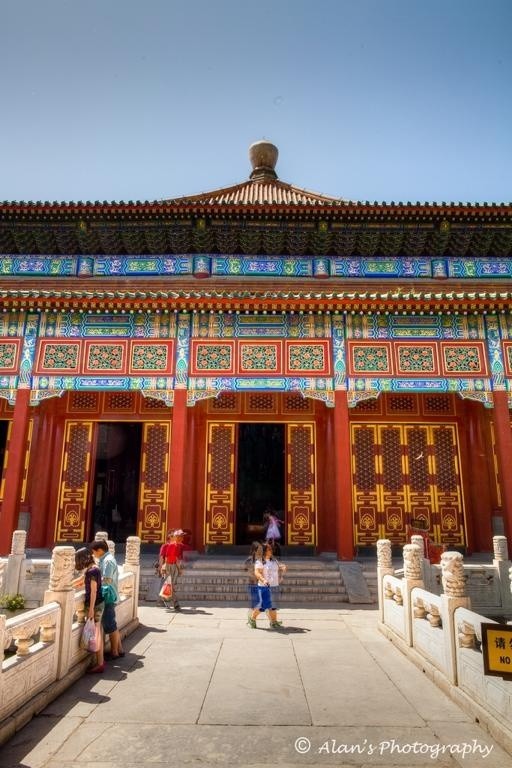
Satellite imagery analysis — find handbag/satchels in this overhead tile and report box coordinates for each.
[101,584,118,605]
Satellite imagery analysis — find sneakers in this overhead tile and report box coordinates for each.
[248,614,283,628]
[85,650,127,675]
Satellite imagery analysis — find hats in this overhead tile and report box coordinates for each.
[173,529,188,537]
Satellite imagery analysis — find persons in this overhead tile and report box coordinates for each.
[70,545,107,674]
[158,528,191,614]
[246,543,286,630]
[263,510,284,545]
[78,538,126,663]
